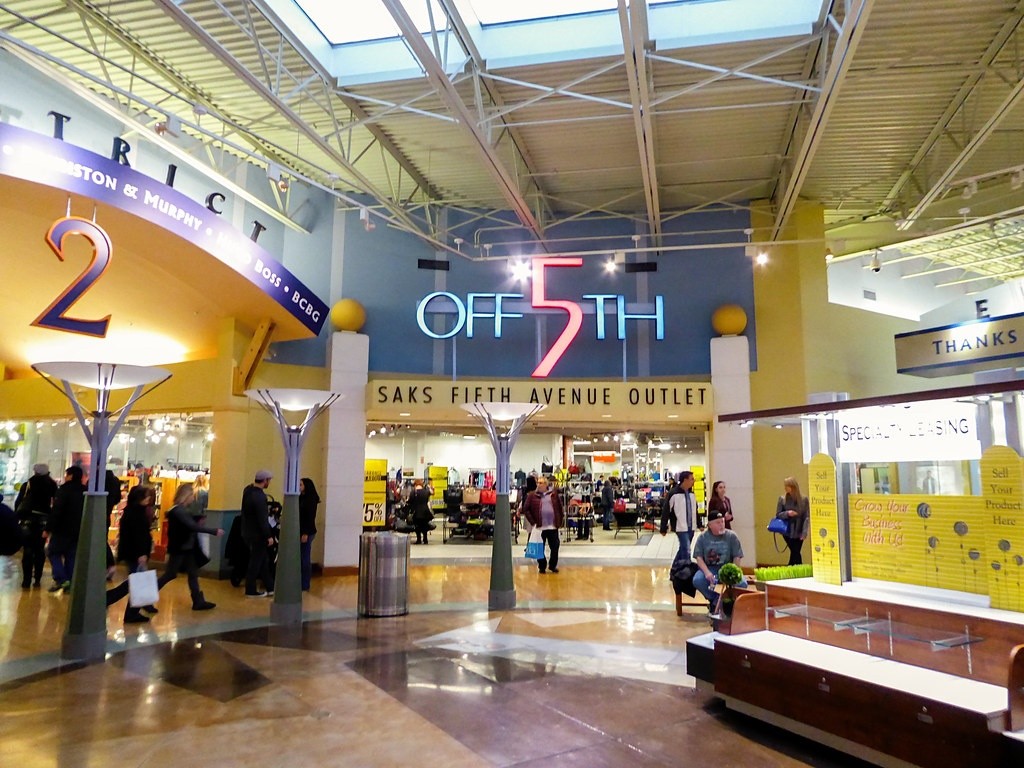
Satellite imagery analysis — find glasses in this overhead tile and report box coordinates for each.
[536,482,544,485]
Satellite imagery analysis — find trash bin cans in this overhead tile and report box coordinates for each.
[357,532,410,616]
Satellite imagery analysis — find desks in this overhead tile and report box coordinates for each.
[647,507,662,532]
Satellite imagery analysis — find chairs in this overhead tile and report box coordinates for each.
[613,512,639,540]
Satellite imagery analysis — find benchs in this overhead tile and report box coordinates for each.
[669,546,711,616]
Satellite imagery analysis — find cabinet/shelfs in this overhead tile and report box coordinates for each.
[687,583,1024,768]
[108,476,195,548]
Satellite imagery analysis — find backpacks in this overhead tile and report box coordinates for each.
[16,481,49,539]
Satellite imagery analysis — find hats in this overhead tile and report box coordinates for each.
[708,510,725,521]
[255,470,272,480]
[32,463,49,476]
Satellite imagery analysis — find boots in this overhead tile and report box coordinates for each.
[191,591,216,610]
[143,605,158,614]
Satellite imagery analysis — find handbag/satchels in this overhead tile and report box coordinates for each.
[767,512,789,553]
[128,561,160,608]
[524,524,545,559]
[198,533,210,559]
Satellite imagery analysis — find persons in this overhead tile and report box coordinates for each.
[405,477,437,545]
[105,485,152,623]
[692,510,741,626]
[523,477,564,573]
[127,459,209,521]
[239,470,276,598]
[14,463,122,595]
[142,487,226,613]
[708,481,733,530]
[575,474,614,540]
[299,478,321,591]
[660,471,704,581]
[776,476,810,564]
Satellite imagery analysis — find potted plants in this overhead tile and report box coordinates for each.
[754,564,813,592]
[717,563,744,636]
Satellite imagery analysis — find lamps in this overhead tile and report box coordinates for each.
[154,117,182,137]
[359,208,369,221]
[870,248,883,272]
[962,187,972,200]
[825,248,833,260]
[1010,178,1021,190]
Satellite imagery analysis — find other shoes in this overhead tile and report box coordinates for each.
[33,581,42,590]
[48,584,63,593]
[539,569,546,574]
[62,581,72,595]
[603,527,613,531]
[124,613,150,624]
[245,590,274,597]
[549,566,559,573]
[20,579,31,591]
[424,541,429,544]
[413,541,422,544]
